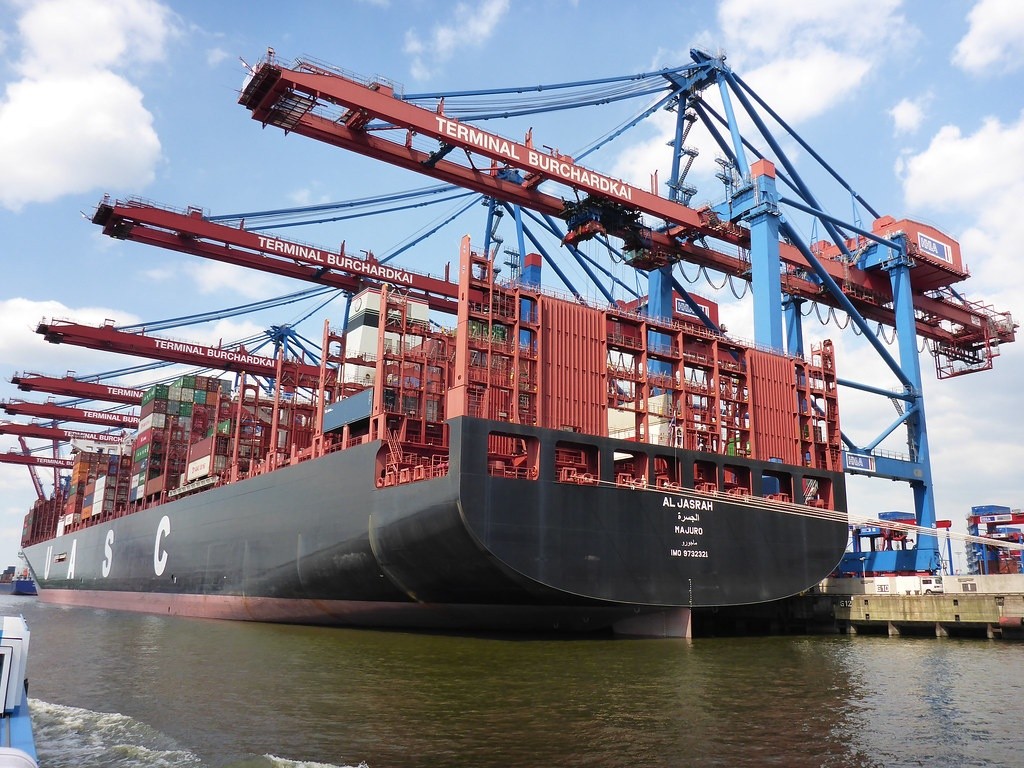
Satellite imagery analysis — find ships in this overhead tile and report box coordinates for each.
[23,286,851,637]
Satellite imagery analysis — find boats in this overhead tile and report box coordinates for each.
[0,613,40,768]
[0,561,37,595]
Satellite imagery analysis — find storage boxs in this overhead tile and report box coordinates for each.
[21,319,507,547]
